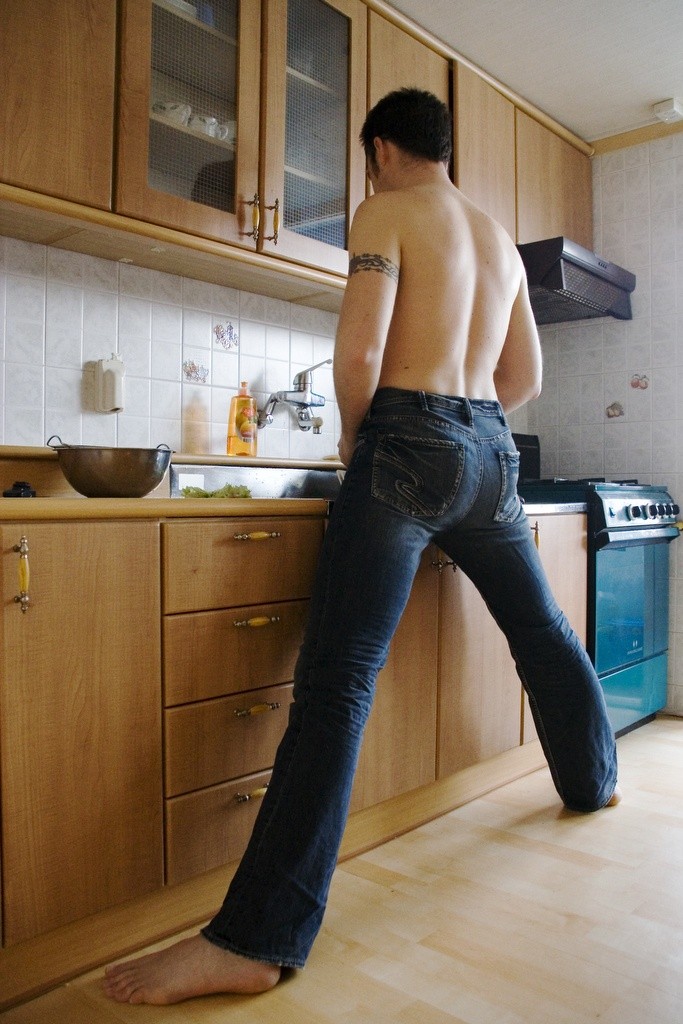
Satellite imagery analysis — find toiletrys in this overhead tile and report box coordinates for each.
[226,382,257,457]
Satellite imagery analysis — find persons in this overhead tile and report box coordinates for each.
[101,88,620,1006]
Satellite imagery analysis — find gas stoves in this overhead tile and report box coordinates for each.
[511,432,668,500]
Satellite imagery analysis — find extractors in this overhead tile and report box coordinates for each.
[515,236,636,325]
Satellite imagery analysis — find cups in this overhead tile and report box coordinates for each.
[222,120,236,143]
[153,102,228,140]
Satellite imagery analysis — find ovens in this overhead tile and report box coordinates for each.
[585,491,681,740]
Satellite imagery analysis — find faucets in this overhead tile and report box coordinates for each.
[258,358,332,434]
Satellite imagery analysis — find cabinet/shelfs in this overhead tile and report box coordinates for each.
[0,497,589,951]
[0,0,595,315]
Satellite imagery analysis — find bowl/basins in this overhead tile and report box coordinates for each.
[46,434,177,498]
